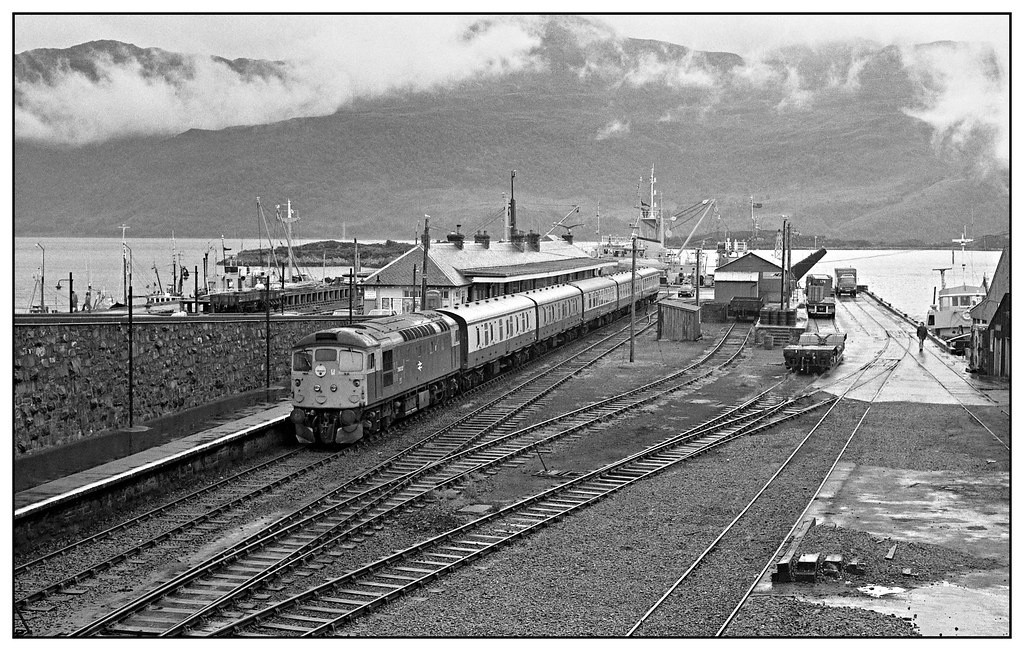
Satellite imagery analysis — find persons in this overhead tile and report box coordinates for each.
[82,286,92,311]
[85,292,92,313]
[72,291,78,312]
[916,322,928,352]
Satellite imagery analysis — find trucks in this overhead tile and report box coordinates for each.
[834,268,857,298]
[806,274,836,319]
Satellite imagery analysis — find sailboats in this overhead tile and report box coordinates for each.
[591,164,718,276]
[191,196,320,301]
[144,230,189,304]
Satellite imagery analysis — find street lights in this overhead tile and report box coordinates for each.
[35,243,44,313]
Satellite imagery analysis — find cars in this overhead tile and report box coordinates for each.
[677,285,695,297]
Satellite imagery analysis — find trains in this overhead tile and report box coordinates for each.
[290,266,661,444]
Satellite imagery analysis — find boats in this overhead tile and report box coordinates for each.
[925,233,988,339]
